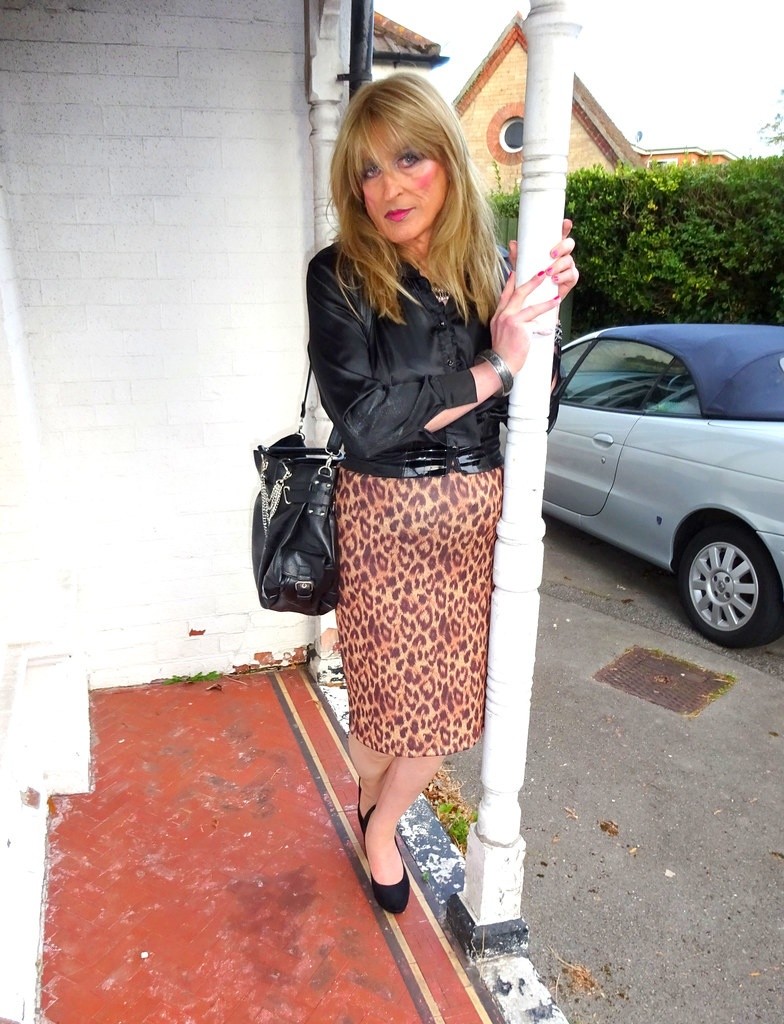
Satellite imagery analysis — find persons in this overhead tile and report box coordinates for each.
[303,74,579,915]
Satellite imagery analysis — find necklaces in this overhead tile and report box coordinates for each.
[430,280,452,303]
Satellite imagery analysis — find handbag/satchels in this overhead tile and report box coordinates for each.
[244,431,348,623]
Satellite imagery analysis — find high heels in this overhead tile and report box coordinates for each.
[357,778,381,827]
[359,809,412,919]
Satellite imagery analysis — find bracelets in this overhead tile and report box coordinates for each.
[472,348,516,399]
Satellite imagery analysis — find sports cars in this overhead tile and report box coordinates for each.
[496,324,784,652]
